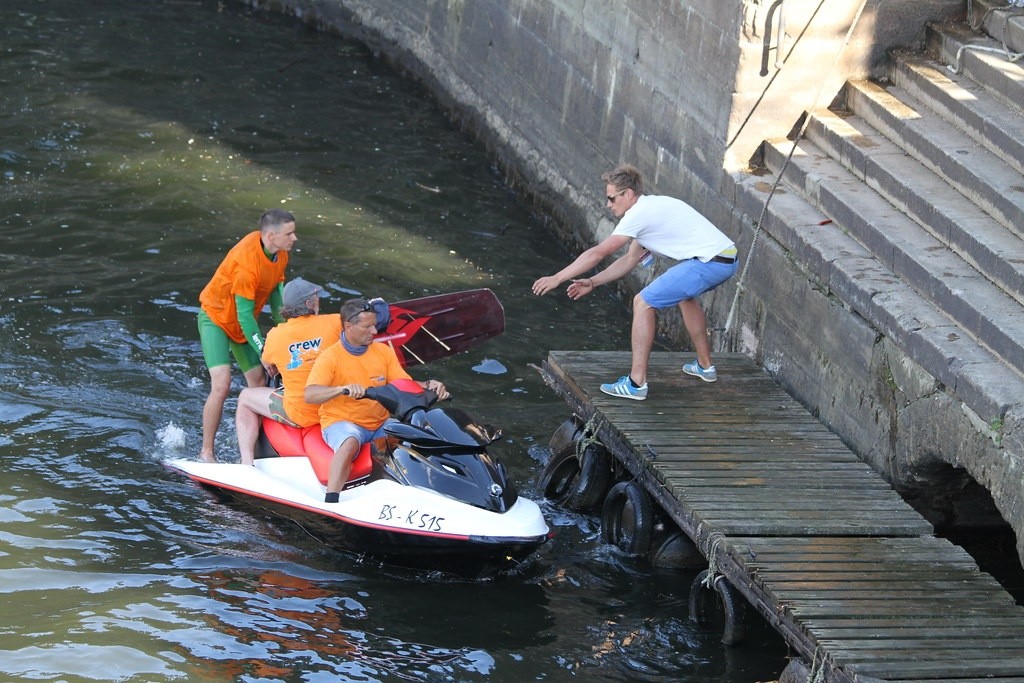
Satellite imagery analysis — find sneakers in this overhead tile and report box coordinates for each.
[682,359,717,382]
[599,374,648,400]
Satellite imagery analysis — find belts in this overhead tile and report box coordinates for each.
[694,255,738,264]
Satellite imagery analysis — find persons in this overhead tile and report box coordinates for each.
[235,277,343,467]
[197,208,299,464]
[303,299,450,503]
[531,165,739,401]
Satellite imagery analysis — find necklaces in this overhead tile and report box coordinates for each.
[341,330,366,355]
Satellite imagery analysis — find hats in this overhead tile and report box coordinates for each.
[282,276,323,309]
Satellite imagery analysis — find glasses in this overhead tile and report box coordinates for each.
[347,304,375,322]
[607,189,627,203]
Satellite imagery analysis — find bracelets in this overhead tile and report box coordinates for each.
[425,379,430,387]
[590,279,596,292]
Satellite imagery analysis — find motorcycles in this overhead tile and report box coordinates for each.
[164,380,558,577]
